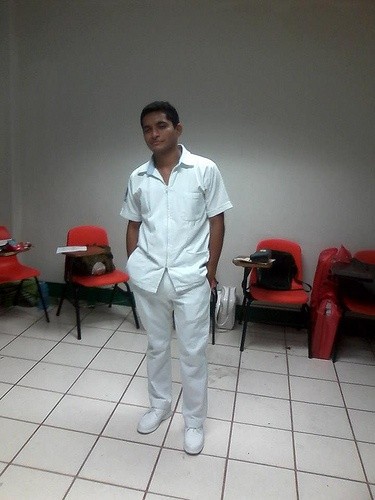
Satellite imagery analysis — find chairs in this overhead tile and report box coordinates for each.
[56,226,140,340]
[0,226,50,323]
[239,239,313,359]
[332,249,375,364]
[210,280,219,345]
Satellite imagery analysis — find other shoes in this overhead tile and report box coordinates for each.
[139,405,172,435]
[183,424,203,456]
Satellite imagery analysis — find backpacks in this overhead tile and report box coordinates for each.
[241,248,313,293]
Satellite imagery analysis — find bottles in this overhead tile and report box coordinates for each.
[36,280,48,310]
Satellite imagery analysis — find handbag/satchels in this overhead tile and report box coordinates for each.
[72,244,114,275]
[343,256,375,303]
[215,286,237,331]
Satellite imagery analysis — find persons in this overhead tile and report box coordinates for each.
[119,101,233,456]
